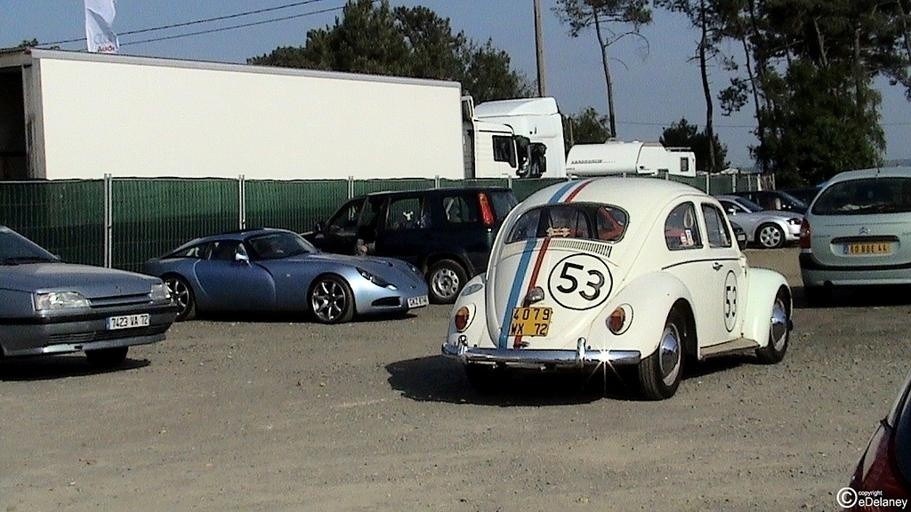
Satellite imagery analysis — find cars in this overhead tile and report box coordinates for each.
[0,166,911,401]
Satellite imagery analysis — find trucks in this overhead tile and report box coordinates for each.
[0,45,532,181]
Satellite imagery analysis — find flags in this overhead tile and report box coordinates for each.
[84,0,121,56]
